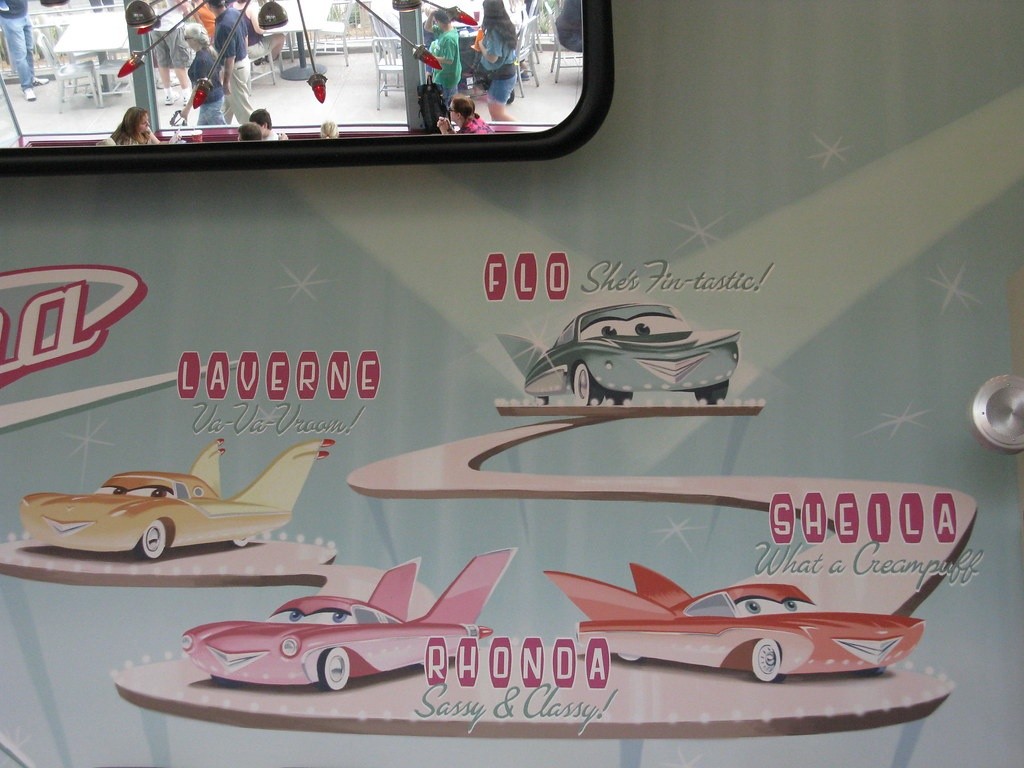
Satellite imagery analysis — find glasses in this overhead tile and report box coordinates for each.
[449,107,455,112]
[185,36,193,40]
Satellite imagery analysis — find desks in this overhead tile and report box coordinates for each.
[452,26,482,87]
[53,10,129,95]
[264,0,332,82]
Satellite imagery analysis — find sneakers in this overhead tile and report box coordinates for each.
[182,91,192,105]
[165,92,180,104]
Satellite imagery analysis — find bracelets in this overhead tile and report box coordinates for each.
[447,128,452,132]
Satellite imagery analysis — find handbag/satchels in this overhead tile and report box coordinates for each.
[473,70,485,84]
[417,75,450,134]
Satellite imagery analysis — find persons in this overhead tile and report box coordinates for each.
[479,0,539,122]
[321,121,338,138]
[0,0,49,100]
[424,5,462,109]
[556,0,583,52]
[148,0,216,105]
[205,0,252,124]
[180,23,226,125]
[111,106,160,145]
[232,0,284,63]
[237,109,289,141]
[437,96,494,134]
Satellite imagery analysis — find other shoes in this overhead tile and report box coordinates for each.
[21,88,36,101]
[266,55,279,62]
[32,79,49,86]
[254,58,263,65]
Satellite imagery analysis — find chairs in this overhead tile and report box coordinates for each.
[249,32,294,88]
[514,0,585,98]
[313,0,355,66]
[36,24,134,114]
[368,12,405,110]
